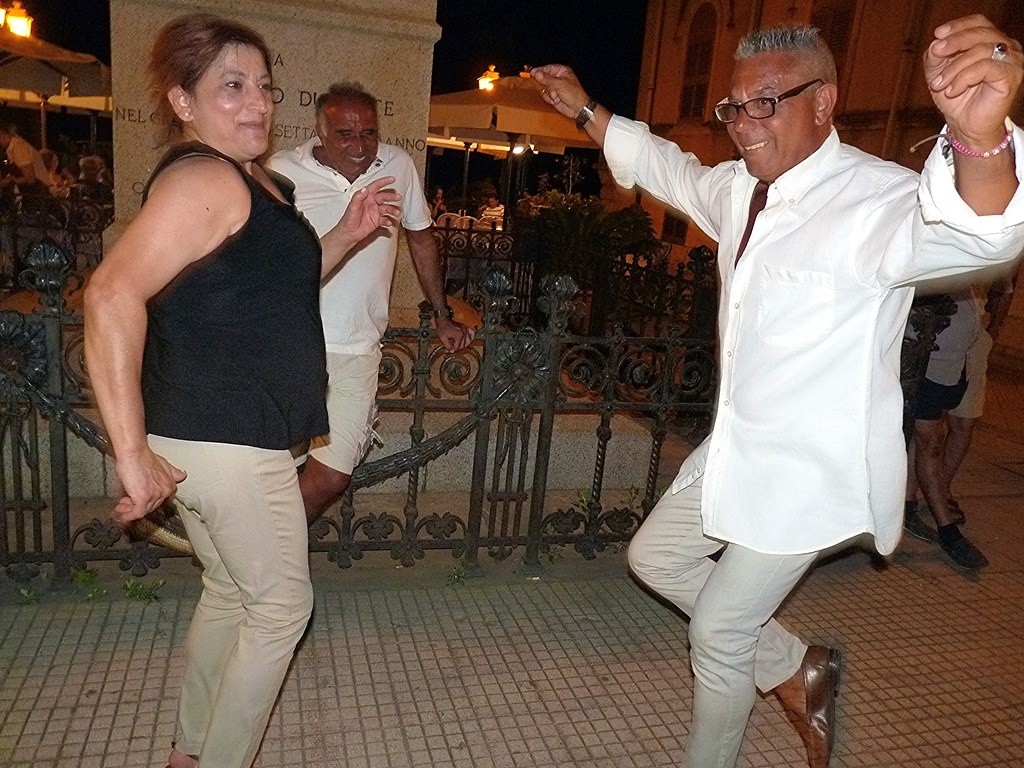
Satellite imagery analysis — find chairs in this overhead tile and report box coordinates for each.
[437,213,461,235]
[454,216,480,234]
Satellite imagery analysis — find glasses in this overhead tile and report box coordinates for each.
[715,77,825,123]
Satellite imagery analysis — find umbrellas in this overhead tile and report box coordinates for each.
[426,75,599,231]
[0,48,113,148]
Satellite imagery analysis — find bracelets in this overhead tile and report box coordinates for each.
[576,97,598,126]
[435,307,454,318]
[909,131,1014,159]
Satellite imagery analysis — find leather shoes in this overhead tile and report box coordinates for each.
[783,645,843,768]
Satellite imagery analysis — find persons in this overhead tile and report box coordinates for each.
[83,22,402,767]
[526,15,1024,768]
[0,119,114,216]
[932,288,993,524]
[428,185,447,224]
[477,194,508,226]
[266,82,475,527]
[900,285,990,568]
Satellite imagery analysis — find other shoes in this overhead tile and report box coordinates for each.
[901,507,938,544]
[946,498,966,523]
[936,527,990,570]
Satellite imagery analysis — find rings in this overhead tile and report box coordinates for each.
[992,42,1008,60]
[541,89,545,95]
